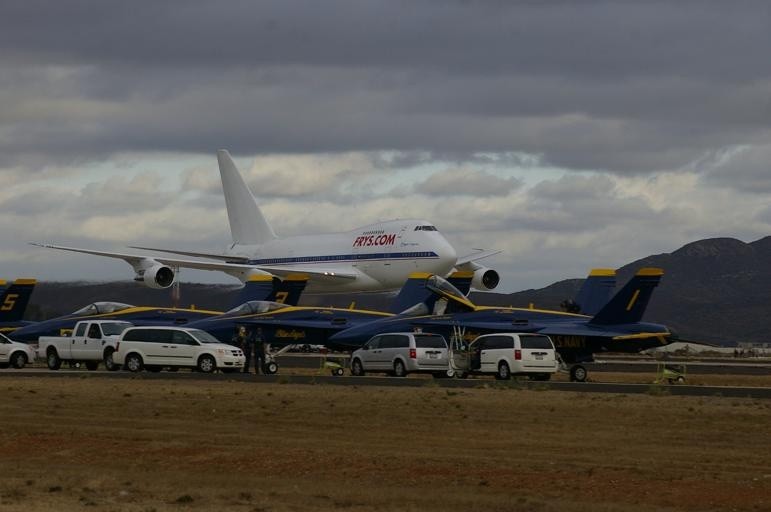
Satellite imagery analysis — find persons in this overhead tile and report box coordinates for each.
[237,326,252,375]
[250,325,267,375]
